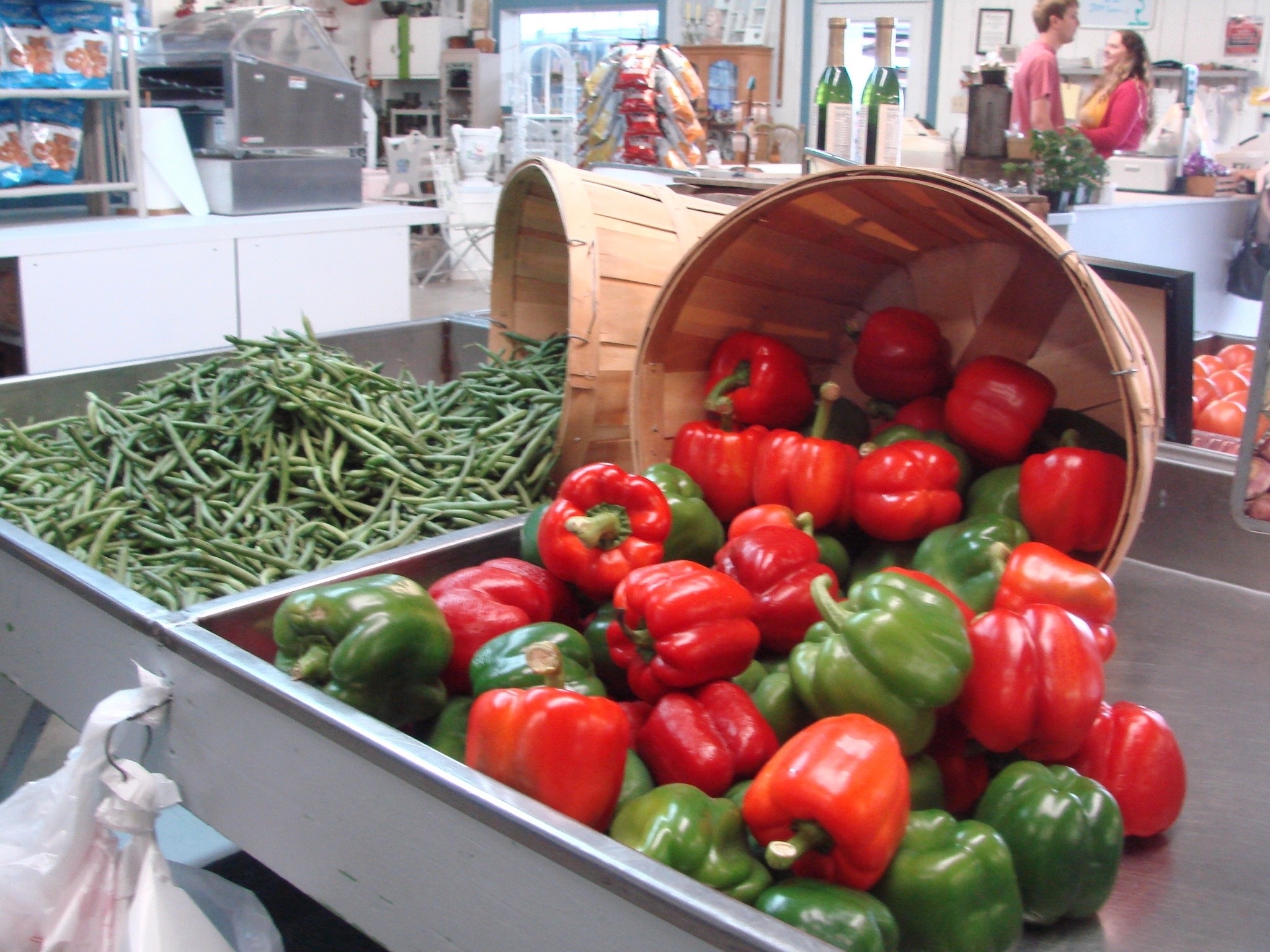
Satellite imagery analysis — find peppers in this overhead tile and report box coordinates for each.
[270,302,1185,952]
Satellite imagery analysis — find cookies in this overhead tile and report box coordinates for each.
[0,131,75,171]
[9,35,107,79]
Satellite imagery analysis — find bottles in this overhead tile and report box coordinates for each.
[856,17,905,166]
[807,18,852,174]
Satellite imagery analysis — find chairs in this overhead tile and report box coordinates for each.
[419,169,501,291]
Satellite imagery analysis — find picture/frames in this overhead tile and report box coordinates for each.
[977,8,1013,55]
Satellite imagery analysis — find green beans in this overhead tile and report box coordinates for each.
[0,310,589,614]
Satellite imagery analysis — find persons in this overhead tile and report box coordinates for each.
[1072,29,1156,161]
[1008,0,1081,211]
[1231,162,1270,272]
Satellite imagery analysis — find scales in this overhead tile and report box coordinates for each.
[1094,63,1199,192]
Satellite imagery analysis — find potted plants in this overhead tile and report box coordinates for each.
[1008,124,1104,211]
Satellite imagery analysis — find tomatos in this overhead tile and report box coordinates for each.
[1189,341,1270,455]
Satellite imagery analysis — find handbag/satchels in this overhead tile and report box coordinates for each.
[1227,183,1270,300]
[1079,85,1111,128]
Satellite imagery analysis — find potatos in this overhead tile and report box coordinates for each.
[1237,436,1270,522]
